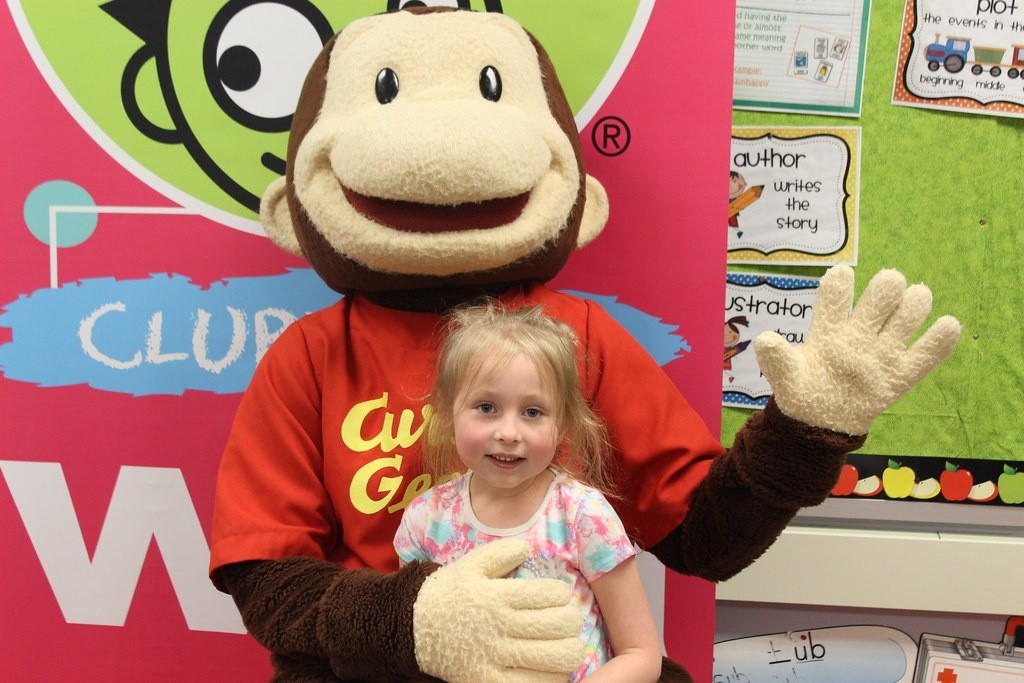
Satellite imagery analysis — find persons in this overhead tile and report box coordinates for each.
[390,302,663,683]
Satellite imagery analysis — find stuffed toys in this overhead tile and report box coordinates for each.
[208,5,965,683]
